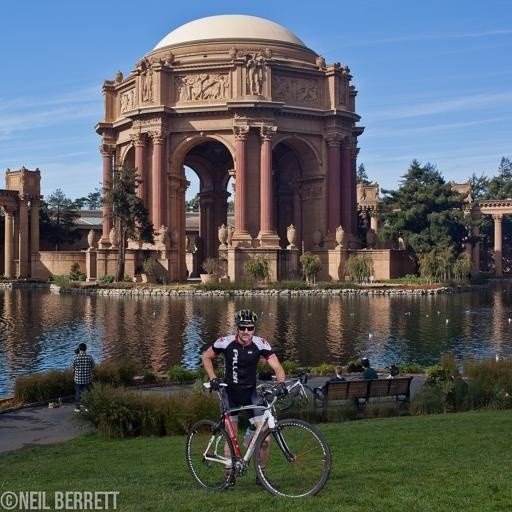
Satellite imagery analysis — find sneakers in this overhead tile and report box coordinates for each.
[256,476,276,486]
[75,407,81,412]
[218,481,236,490]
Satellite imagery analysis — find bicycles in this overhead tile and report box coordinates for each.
[256,369,310,411]
[184,381,332,498]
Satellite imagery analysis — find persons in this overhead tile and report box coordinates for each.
[317,365,346,395]
[201,308,287,486]
[72,343,96,412]
[360,358,380,379]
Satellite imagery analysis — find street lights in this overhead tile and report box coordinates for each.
[56,203,66,250]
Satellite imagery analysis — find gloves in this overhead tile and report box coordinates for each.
[272,382,286,400]
[209,377,224,391]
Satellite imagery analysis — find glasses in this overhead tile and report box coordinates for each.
[237,325,255,331]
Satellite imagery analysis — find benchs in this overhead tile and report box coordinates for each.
[314,376,415,410]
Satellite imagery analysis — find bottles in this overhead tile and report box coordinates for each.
[242,423,257,447]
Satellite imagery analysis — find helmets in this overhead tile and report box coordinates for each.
[234,309,257,325]
